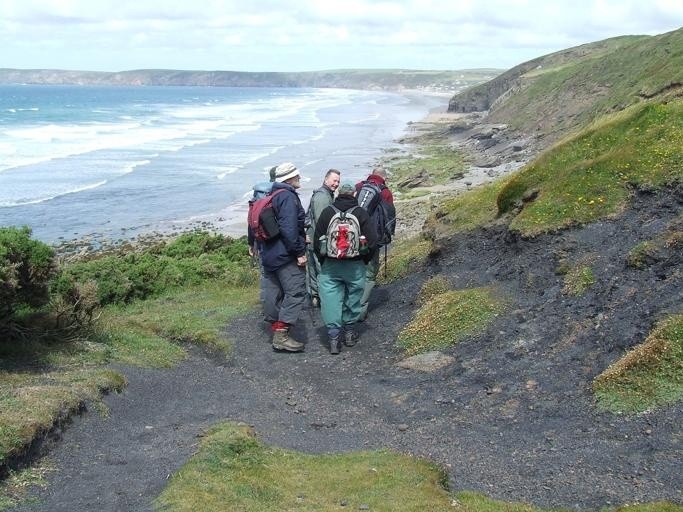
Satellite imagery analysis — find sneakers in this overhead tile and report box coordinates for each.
[311,297,319,307]
[344,325,359,346]
[272,329,304,351]
[329,336,342,354]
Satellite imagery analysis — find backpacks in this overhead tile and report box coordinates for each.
[301,188,331,228]
[248,189,288,239]
[356,181,387,216]
[326,205,361,258]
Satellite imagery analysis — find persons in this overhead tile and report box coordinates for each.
[352,168,396,281]
[262,163,308,353]
[313,183,377,353]
[247,165,280,317]
[306,169,340,309]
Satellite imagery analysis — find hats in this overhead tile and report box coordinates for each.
[275,163,300,183]
[270,166,278,182]
[373,169,387,178]
